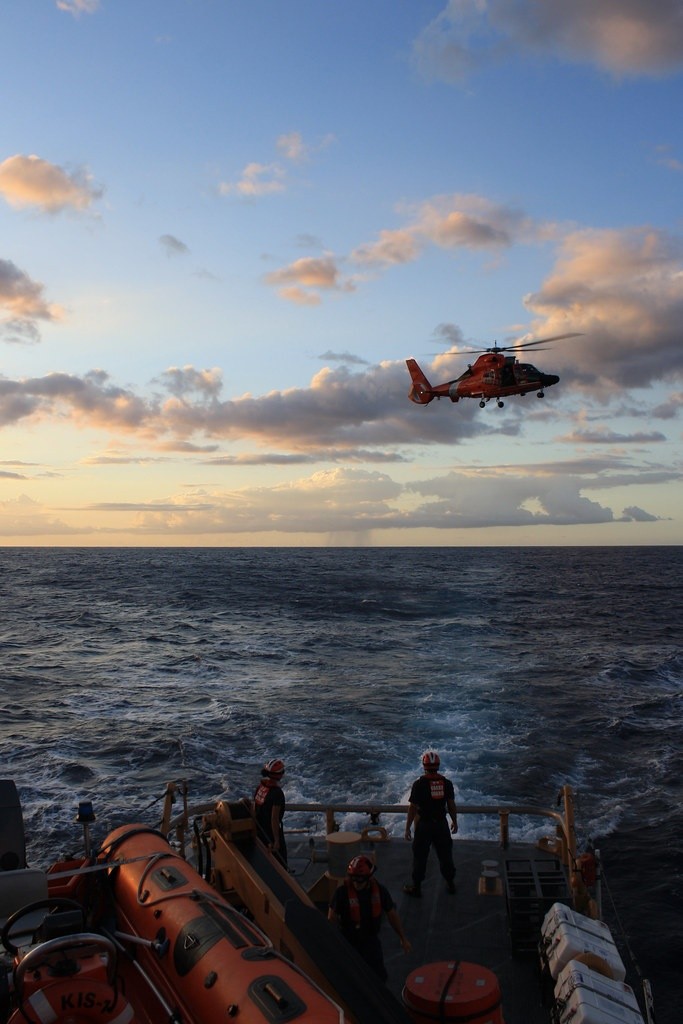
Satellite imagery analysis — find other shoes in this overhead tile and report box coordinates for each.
[446,882,456,895]
[403,884,421,898]
[285,868,295,873]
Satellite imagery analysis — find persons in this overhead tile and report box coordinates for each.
[328,856,411,983]
[254,759,297,874]
[404,752,458,897]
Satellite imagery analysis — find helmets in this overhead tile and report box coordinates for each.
[264,758,285,772]
[423,752,440,770]
[347,856,374,875]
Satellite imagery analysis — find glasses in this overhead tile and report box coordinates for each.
[278,769,285,774]
[352,876,367,883]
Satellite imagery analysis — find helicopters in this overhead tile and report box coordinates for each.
[407,331,587,409]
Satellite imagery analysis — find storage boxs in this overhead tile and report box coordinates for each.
[540,903,626,982]
[554,960,645,1024]
